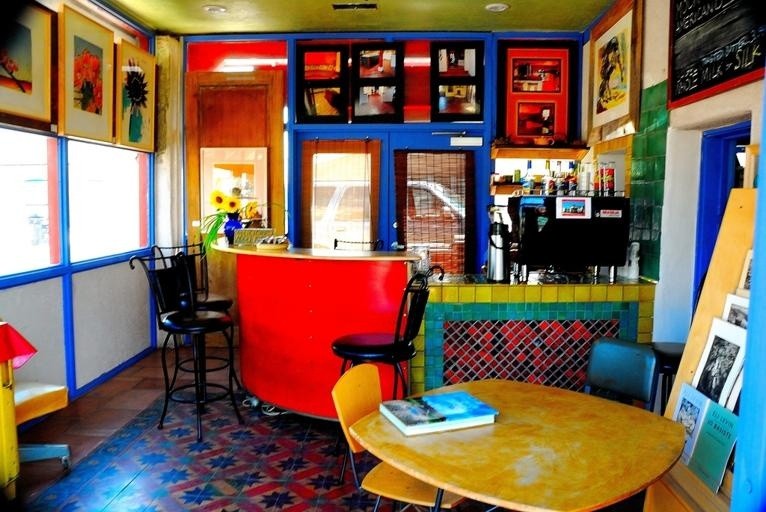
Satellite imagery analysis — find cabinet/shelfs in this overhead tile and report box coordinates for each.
[490,147,588,196]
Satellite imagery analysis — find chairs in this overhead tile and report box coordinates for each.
[584,337,659,413]
[641,272,708,416]
[329,264,444,455]
[129,253,245,442]
[151,243,242,393]
[331,364,466,512]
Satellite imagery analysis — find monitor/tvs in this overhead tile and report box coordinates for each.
[519,197,630,269]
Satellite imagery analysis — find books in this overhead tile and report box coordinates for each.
[672,383,708,467]
[379,390,502,438]
[688,401,739,496]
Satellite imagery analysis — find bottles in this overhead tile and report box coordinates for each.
[523,157,615,196]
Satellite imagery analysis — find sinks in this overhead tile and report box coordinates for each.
[429,274,478,284]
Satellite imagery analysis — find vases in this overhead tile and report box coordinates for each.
[224,212,241,245]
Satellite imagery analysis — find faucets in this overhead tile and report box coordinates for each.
[421,264,445,280]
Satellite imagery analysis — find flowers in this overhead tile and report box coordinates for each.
[193,191,258,251]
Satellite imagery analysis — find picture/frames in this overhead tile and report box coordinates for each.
[115,37,156,153]
[586,0,644,148]
[57,2,114,143]
[0,0,59,134]
[666,0,766,112]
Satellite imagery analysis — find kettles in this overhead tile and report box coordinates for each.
[489,222,511,282]
